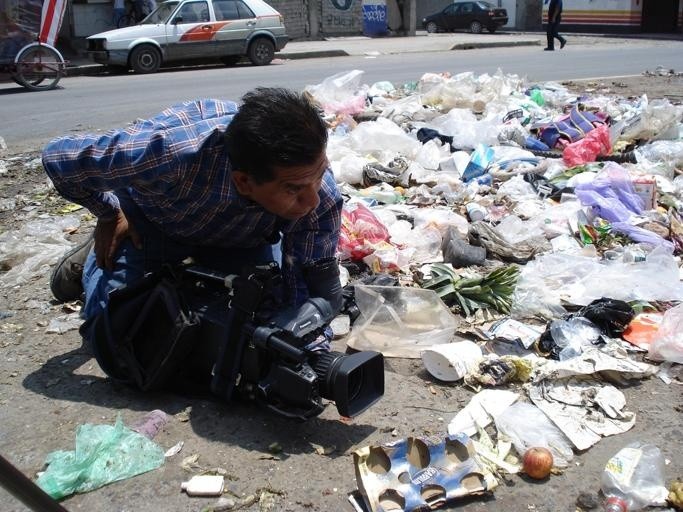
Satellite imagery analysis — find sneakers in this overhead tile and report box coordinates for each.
[544,39,566,50]
[50,228,95,302]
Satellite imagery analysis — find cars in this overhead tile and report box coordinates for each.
[83,0,288,74]
[421,1,508,34]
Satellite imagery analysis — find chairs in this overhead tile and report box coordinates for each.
[180,5,208,21]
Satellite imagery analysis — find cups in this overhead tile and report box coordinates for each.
[420,340,482,381]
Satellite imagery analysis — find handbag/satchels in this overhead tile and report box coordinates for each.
[107,273,202,391]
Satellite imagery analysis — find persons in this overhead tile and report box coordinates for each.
[544,0,566,51]
[41,86,343,419]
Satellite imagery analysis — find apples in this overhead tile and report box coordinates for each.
[524,447,553,480]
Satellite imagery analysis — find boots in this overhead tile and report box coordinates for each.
[442,225,487,269]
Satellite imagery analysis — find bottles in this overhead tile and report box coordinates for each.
[599,441,665,511]
